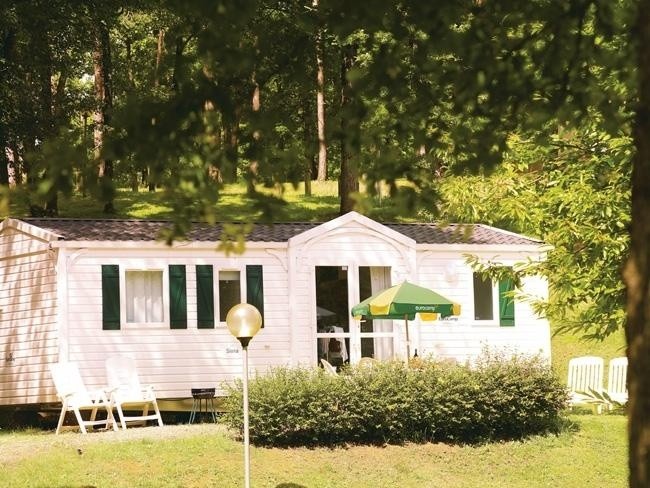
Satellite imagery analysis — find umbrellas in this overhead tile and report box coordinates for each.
[317,306,338,326]
[349,279,462,371]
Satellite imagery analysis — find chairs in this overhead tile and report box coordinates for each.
[50,356,164,434]
[565,356,629,414]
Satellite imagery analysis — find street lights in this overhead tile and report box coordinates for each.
[223,301,265,488]
